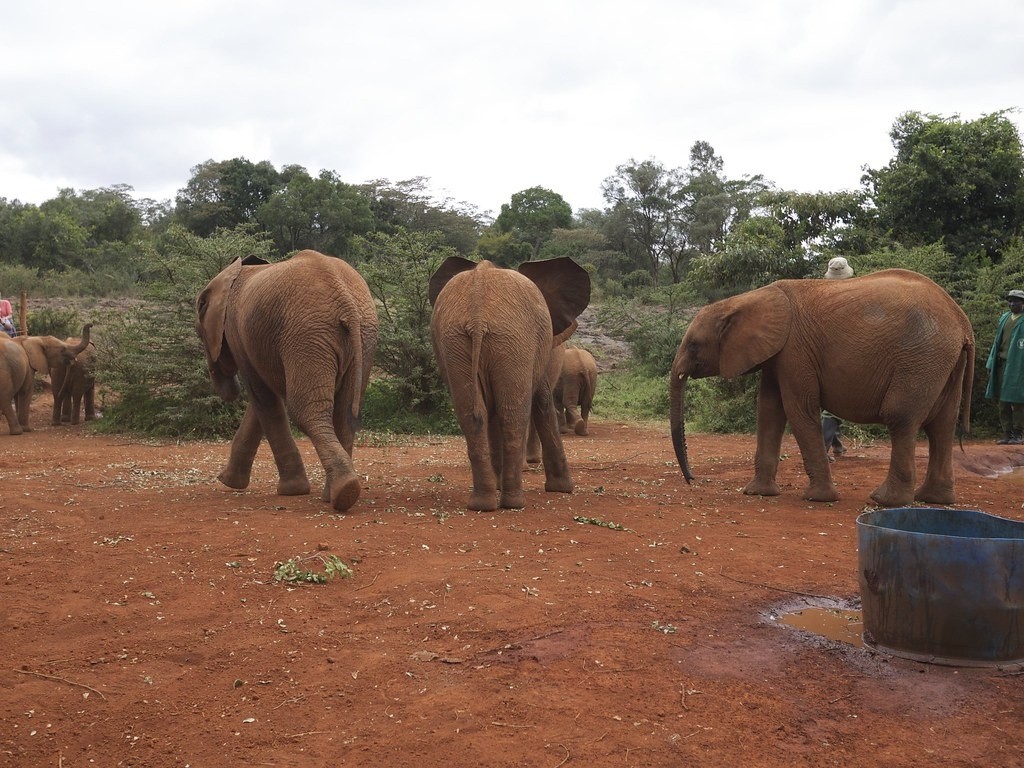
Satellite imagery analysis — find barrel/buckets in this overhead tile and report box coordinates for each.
[855,508,1024,668]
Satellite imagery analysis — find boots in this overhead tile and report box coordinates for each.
[832,417,847,455]
[1008,408,1024,445]
[821,417,837,463]
[996,408,1012,444]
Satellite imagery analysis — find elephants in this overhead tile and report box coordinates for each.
[668,268,976,509]
[428,247,599,511]
[0,322,98,435]
[194,250,379,513]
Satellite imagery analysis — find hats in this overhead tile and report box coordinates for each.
[1006,290,1024,301]
[824,257,854,279]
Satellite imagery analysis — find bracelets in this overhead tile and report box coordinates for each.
[2,322,6,326]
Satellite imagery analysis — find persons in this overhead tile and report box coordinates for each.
[985,291,1024,445]
[0,300,16,339]
[820,257,856,463]
